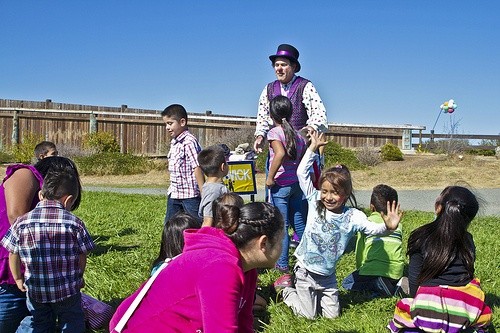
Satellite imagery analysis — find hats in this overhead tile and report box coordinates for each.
[269,44,302,73]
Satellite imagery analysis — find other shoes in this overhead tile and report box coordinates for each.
[274,263,290,273]
[289,231,300,249]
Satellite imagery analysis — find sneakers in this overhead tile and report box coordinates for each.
[393,276,410,299]
[270,274,293,296]
[376,276,395,298]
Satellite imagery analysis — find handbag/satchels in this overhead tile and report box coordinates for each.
[79,292,116,332]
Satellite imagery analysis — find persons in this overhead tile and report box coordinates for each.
[386,185,492,333]
[0,168,97,333]
[271,130,403,319]
[212,190,267,310]
[34,141,58,162]
[254,43,328,244]
[160,104,205,226]
[0,156,82,333]
[265,95,306,273]
[198,146,231,228]
[109,202,285,333]
[150,212,199,276]
[348,184,404,297]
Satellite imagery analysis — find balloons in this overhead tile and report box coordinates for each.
[440,99,457,113]
